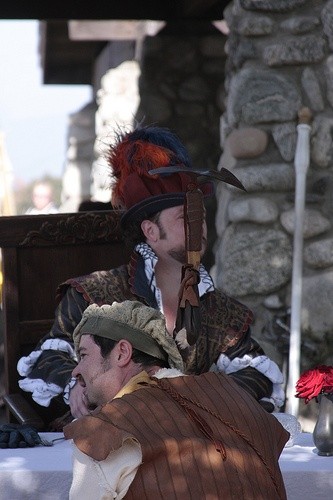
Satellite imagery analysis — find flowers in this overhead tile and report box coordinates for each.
[295,366,333,404]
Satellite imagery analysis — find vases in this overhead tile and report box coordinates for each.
[313,392,333,457]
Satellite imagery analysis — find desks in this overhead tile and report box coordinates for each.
[0,432,333,500]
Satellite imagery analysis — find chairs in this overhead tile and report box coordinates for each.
[0,210,136,449]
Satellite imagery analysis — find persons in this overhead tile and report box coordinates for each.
[16,128,284,500]
[61,300,291,500]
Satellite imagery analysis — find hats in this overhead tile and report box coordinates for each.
[108,122,214,228]
[73,300,185,370]
